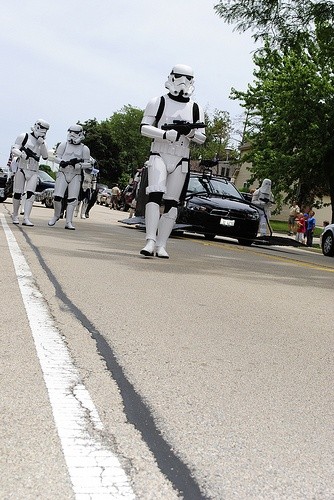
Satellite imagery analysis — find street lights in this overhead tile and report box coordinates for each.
[224,147,232,176]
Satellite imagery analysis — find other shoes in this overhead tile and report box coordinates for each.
[85,212,89,218]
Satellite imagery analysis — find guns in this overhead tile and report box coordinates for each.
[161,120,208,141]
[259,198,276,204]
[19,146,39,162]
[66,158,84,169]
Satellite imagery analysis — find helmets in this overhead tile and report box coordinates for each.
[164,64,196,99]
[260,178,272,194]
[30,119,49,139]
[89,156,95,170]
[67,125,84,145]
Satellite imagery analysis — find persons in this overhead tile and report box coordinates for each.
[289,200,316,246]
[252,179,274,240]
[11,119,48,226]
[110,184,120,209]
[140,64,207,259]
[75,155,97,218]
[129,161,149,219]
[49,125,91,230]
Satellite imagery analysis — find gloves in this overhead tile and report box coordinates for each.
[72,164,80,170]
[37,137,44,144]
[60,161,67,168]
[267,202,272,207]
[165,129,179,142]
[260,202,266,206]
[182,126,195,138]
[22,154,28,160]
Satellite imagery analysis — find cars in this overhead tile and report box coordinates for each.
[35,170,56,208]
[171,170,260,246]
[96,184,134,211]
[319,224,334,256]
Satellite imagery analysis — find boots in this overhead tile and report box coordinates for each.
[139,202,161,256]
[156,206,178,259]
[47,199,61,226]
[65,199,75,230]
[80,198,88,219]
[128,198,137,218]
[74,200,79,217]
[11,198,20,224]
[22,195,35,226]
